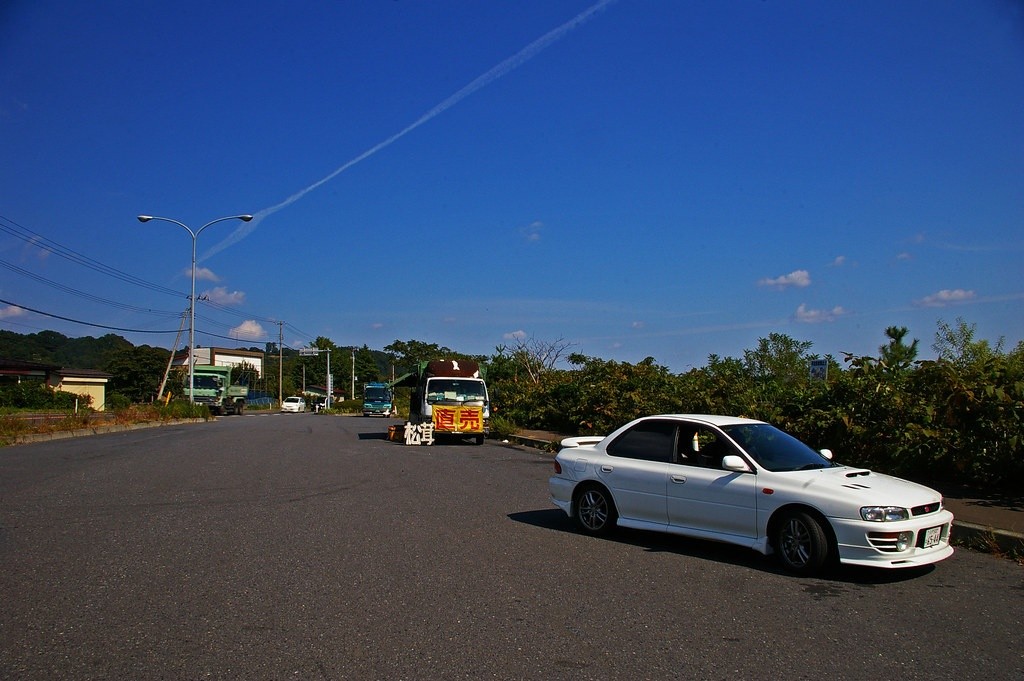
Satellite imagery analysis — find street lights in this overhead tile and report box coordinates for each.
[135,214,255,406]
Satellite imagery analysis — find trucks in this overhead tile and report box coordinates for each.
[182,365,249,415]
[362,383,393,418]
[408,359,491,445]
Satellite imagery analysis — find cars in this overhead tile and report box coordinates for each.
[281,397,307,414]
[549,414,954,577]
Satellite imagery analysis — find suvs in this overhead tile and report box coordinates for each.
[311,395,334,412]
